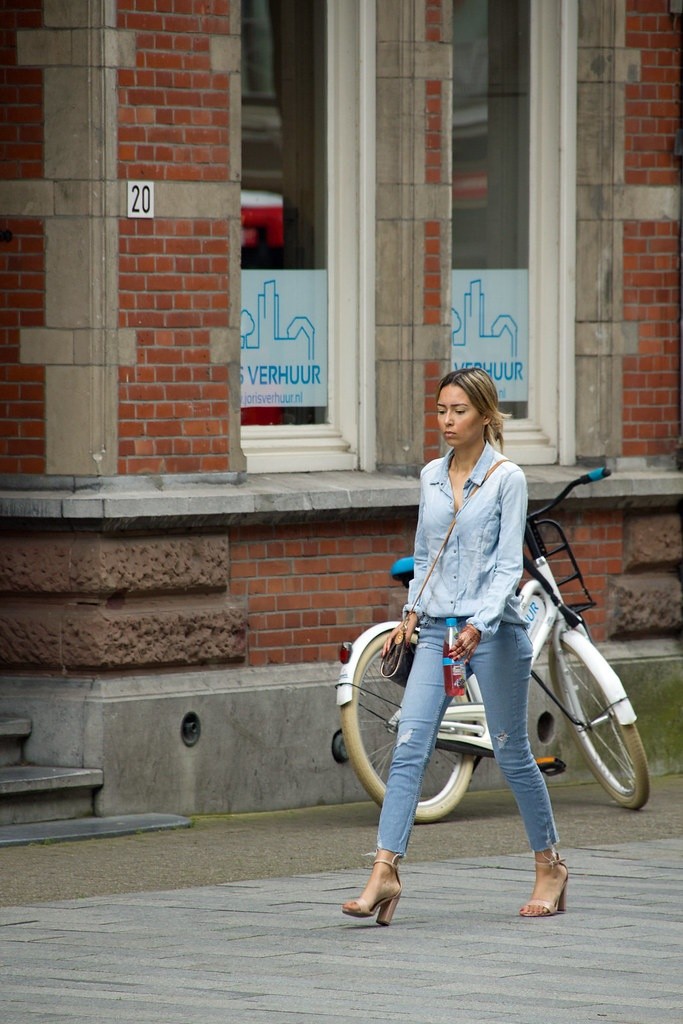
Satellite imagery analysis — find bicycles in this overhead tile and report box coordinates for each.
[334,469,650,822]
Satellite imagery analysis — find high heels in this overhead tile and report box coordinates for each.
[341,854,404,926]
[519,851,570,917]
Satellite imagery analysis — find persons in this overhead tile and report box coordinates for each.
[342,369,569,925]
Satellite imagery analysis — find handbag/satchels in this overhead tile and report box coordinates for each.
[379,627,419,687]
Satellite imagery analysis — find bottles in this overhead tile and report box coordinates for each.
[442,619,465,696]
